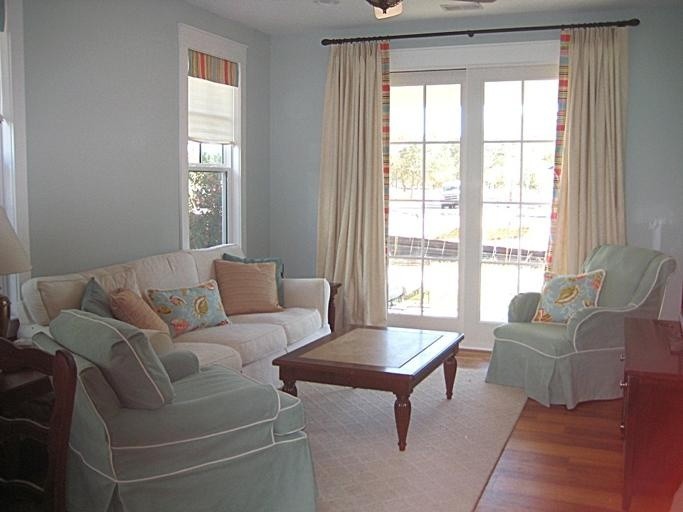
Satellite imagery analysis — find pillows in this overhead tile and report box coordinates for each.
[144,281,230,338]
[111,285,169,336]
[213,259,284,314]
[222,252,284,306]
[79,277,111,316]
[528,268,605,324]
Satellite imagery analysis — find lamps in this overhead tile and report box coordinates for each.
[0,208,34,340]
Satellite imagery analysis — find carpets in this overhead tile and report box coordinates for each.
[229,369,529,511]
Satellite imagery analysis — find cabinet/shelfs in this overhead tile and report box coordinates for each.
[614,316,682,512]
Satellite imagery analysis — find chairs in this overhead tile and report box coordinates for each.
[0,337,78,509]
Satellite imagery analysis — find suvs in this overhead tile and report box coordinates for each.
[440,181,460,209]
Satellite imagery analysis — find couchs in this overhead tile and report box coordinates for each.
[18,242,342,389]
[33,308,317,512]
[485,244,677,409]
